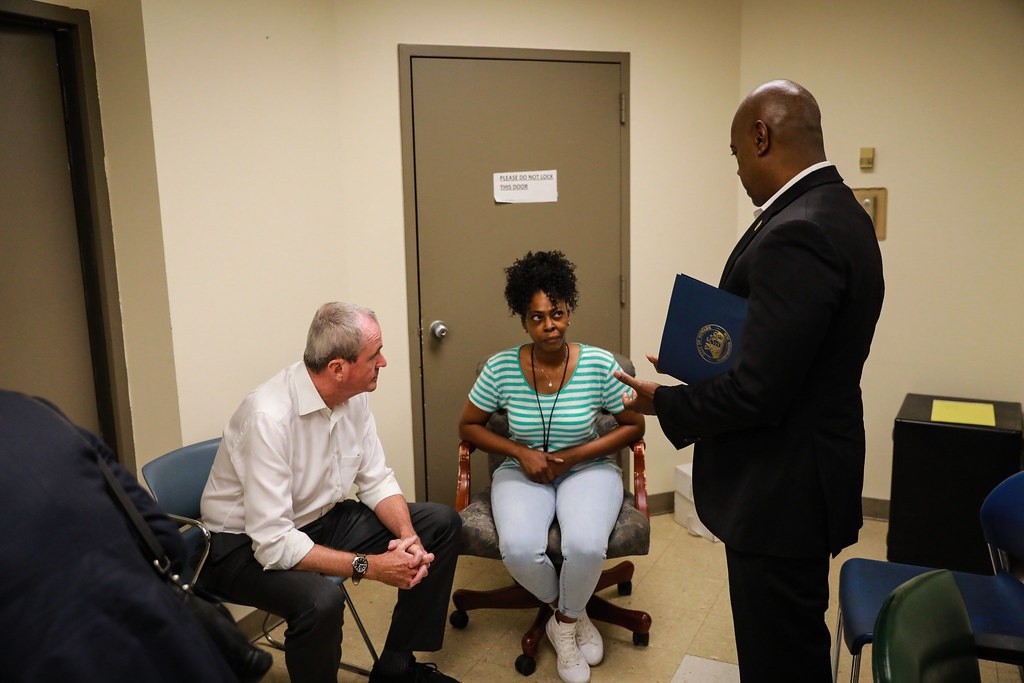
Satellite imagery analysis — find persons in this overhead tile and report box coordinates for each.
[458,250,645,683]
[609,72,886,683]
[198,301,461,683]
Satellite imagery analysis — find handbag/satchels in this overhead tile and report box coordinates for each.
[176,585,272,682]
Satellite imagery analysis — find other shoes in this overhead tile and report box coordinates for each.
[368,658,463,683]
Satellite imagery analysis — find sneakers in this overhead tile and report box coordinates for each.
[545,603,603,682]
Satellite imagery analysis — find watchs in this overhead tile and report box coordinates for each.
[353,552,368,581]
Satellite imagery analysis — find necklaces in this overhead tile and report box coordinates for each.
[531,349,568,387]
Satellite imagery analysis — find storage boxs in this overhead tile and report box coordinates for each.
[671,460,722,543]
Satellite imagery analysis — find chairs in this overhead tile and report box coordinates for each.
[871,570,983,683]
[833,469,1023,683]
[140,438,377,677]
[448,352,651,675]
